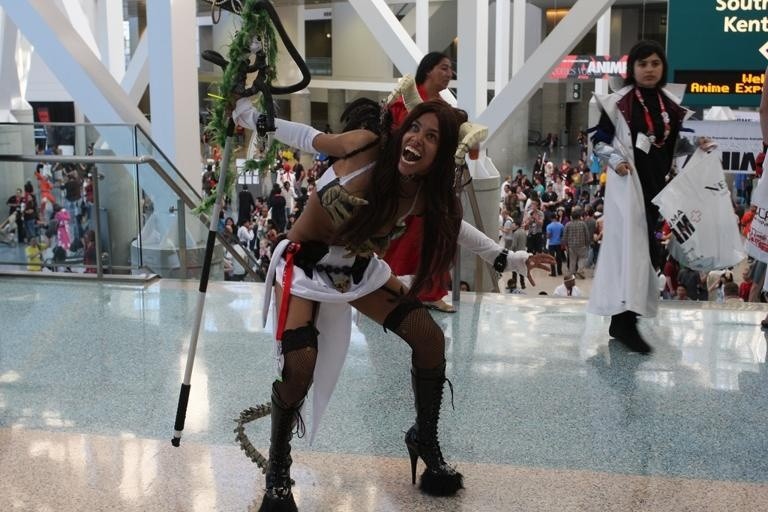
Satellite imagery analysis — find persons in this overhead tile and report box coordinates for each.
[378,51,459,315]
[225,89,556,512]
[757,62,768,330]
[199,123,343,282]
[489,146,768,306]
[583,38,714,356]
[0,133,113,276]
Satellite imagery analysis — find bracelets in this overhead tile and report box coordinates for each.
[256,114,271,136]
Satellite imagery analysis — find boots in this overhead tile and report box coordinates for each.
[403,358,462,497]
[260,381,308,512]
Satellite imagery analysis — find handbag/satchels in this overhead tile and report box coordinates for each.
[649,145,746,271]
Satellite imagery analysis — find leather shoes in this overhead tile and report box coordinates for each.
[609,312,651,355]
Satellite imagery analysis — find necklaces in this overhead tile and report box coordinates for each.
[633,86,671,150]
